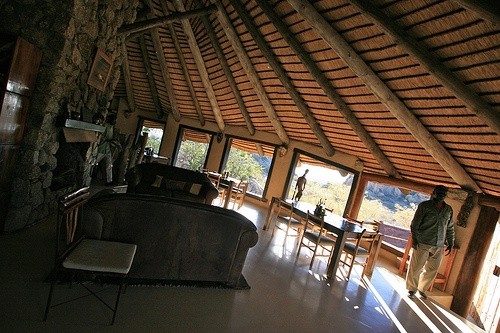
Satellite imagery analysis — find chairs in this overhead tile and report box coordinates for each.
[271,193,381,279]
[42,184,137,325]
[202,163,248,208]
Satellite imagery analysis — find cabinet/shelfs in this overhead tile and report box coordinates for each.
[397,231,460,293]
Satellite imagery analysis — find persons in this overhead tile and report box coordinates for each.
[407,186,455,299]
[291,169,310,201]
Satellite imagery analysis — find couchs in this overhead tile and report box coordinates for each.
[125,162,219,205]
[80,187,259,288]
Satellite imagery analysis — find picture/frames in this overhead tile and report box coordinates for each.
[85,46,114,92]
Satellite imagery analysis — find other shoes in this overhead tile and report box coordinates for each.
[408,291,416,299]
[419,291,427,299]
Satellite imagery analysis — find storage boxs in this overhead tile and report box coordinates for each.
[0,35,42,175]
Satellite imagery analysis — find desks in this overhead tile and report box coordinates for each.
[199,159,249,208]
[263,186,387,276]
